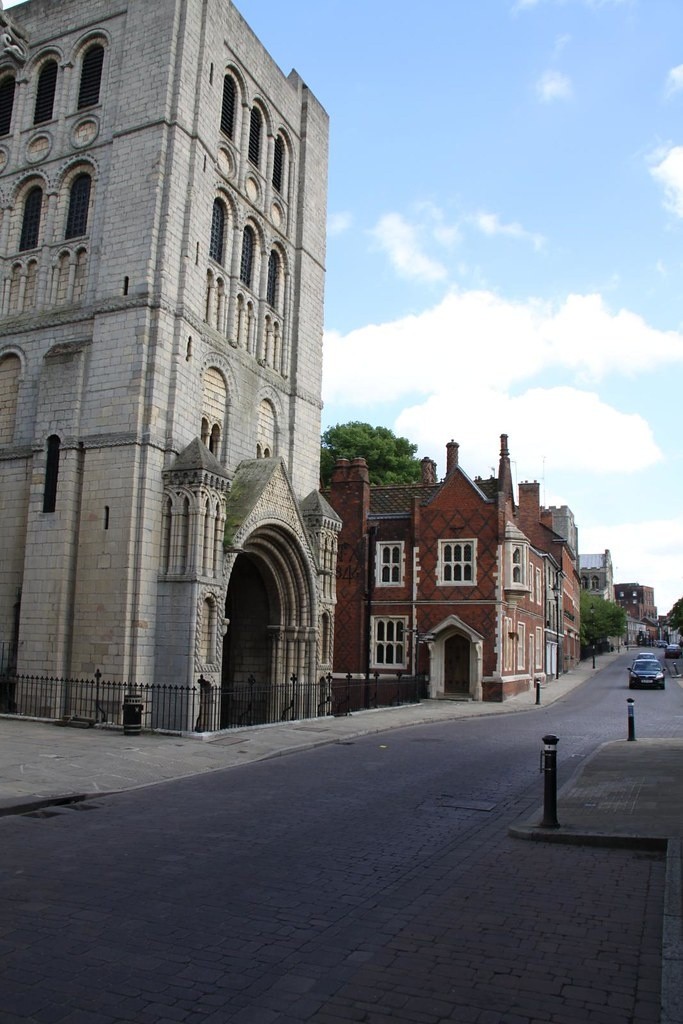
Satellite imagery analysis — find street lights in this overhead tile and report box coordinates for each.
[552,584,561,679]
[590,603,597,669]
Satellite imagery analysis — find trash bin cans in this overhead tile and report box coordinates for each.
[122,693,144,736]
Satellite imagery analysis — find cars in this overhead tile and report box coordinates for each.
[665,643,682,658]
[634,653,656,661]
[653,640,669,647]
[627,660,666,690]
